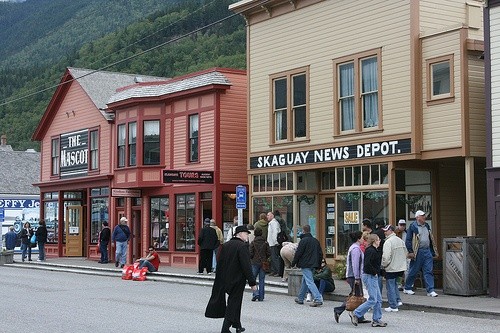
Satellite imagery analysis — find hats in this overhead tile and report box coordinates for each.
[381,224,395,231]
[234,226,251,236]
[399,219,406,224]
[120,217,128,221]
[204,218,210,225]
[415,210,426,218]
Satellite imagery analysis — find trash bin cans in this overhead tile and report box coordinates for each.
[443,237,485,296]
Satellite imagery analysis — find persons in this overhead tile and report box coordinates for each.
[35,220,48,262]
[349,233,387,327]
[358,218,416,299]
[112,217,131,268]
[97,221,111,264]
[203,226,258,333]
[20,222,33,262]
[305,257,336,302]
[381,225,408,312]
[209,219,224,273]
[196,221,218,275]
[333,232,372,324]
[139,246,160,272]
[291,225,323,307]
[227,210,293,278]
[250,228,272,302]
[403,210,439,297]
[4,226,18,263]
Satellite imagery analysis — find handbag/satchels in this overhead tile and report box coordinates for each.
[205,277,226,318]
[345,283,365,311]
[132,267,148,281]
[122,265,134,280]
[262,262,269,270]
[29,243,37,247]
[30,232,36,243]
[277,231,288,245]
[133,262,140,270]
[96,245,101,253]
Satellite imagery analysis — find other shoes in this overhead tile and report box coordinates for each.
[37,257,44,261]
[115,261,119,267]
[28,259,32,261]
[101,261,108,264]
[121,264,124,268]
[98,261,102,263]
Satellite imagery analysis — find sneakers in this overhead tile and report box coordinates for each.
[196,269,438,333]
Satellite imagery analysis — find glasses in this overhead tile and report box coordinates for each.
[149,249,153,251]
[321,261,324,263]
